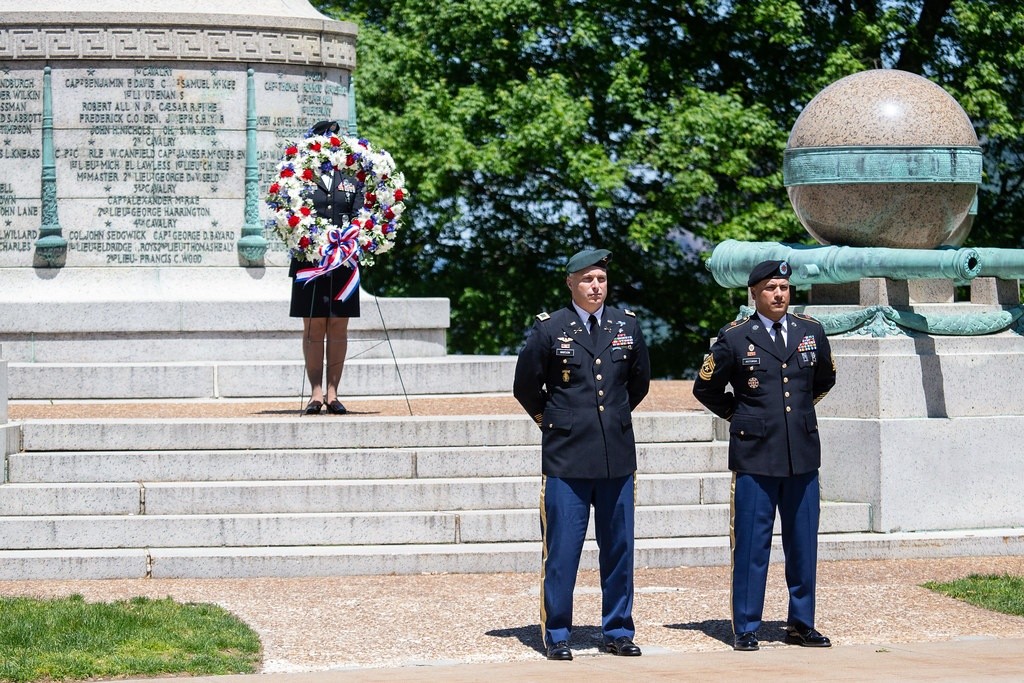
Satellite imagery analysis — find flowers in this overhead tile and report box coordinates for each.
[265,125,410,270]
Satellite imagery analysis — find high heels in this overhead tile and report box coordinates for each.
[305,394,325,414]
[323,395,347,414]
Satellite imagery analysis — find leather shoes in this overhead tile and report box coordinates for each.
[734,632,759,650]
[546,640,573,661]
[785,624,830,648]
[604,636,641,656]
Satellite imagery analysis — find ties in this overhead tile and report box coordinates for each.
[588,314,599,347]
[772,322,786,359]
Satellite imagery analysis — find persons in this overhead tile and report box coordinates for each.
[691,259,837,651]
[512,248,650,661]
[286,120,370,415]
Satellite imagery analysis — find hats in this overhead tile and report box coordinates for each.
[566,249,613,273]
[747,260,792,287]
[310,120,340,136]
